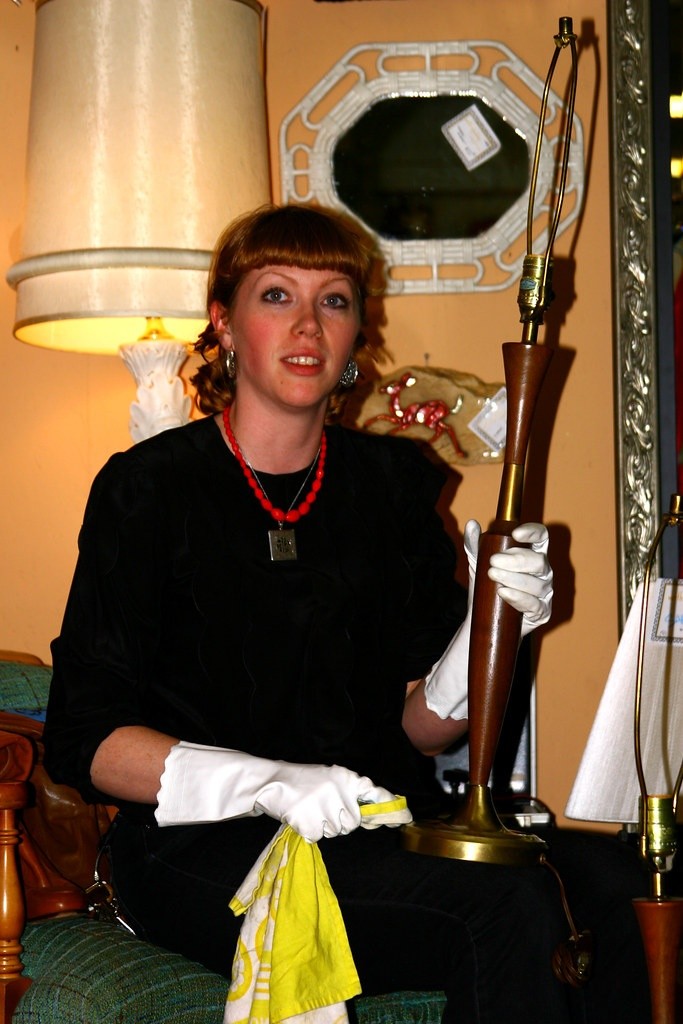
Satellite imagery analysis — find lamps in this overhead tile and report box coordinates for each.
[6,1,271,446]
[571,581,683,825]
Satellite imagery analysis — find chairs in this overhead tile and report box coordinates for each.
[1,651,125,1024]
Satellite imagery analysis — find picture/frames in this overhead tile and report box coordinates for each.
[606,1,683,647]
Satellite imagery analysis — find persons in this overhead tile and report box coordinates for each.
[38,205,662,1024]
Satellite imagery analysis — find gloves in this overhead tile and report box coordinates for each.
[424,518,554,720]
[154,740,402,842]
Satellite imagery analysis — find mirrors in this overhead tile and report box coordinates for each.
[279,40,583,293]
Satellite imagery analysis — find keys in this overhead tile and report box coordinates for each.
[84,879,137,936]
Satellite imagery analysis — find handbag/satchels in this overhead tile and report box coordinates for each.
[0,711,112,922]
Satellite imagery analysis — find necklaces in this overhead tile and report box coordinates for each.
[223,404,327,562]
